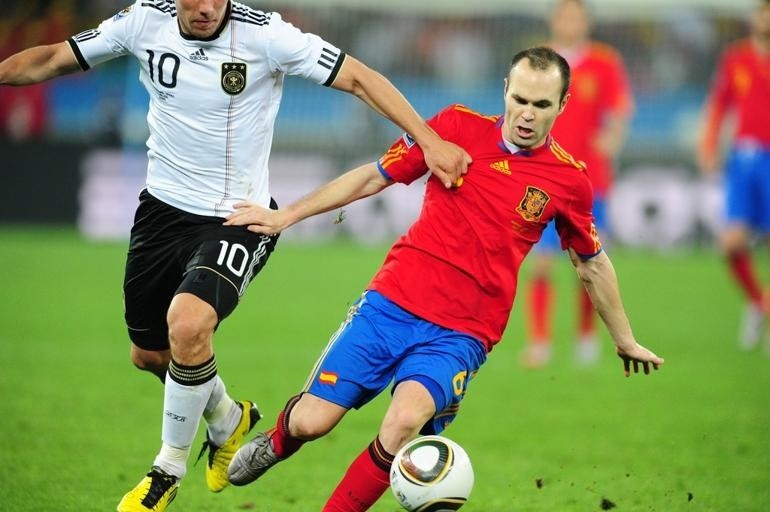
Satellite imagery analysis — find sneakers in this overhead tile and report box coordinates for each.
[116,465,179,512]
[227,425,282,486]
[193,399,261,493]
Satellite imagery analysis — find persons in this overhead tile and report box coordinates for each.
[2,0,472,512]
[696,0,769,354]
[222,45,667,512]
[523,0,635,367]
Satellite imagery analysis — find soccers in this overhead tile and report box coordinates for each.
[389,435,474,507]
